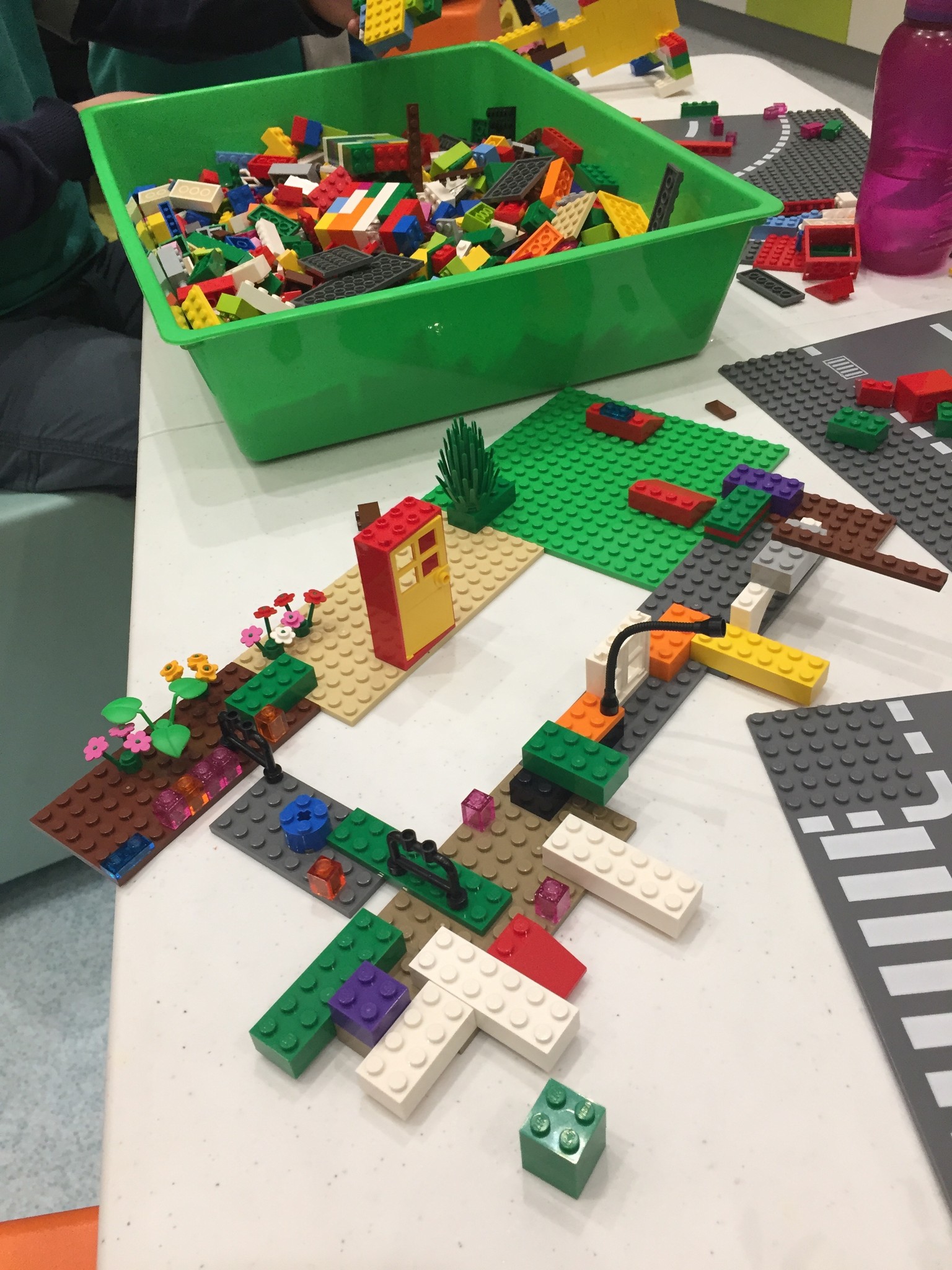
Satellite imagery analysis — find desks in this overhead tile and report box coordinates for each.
[95,0,952,1270]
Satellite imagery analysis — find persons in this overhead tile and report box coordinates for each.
[78,12,381,123]
[1,1,379,502]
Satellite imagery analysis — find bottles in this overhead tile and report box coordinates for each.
[855,0,952,275]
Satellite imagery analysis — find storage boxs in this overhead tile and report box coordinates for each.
[75,38,784,469]
